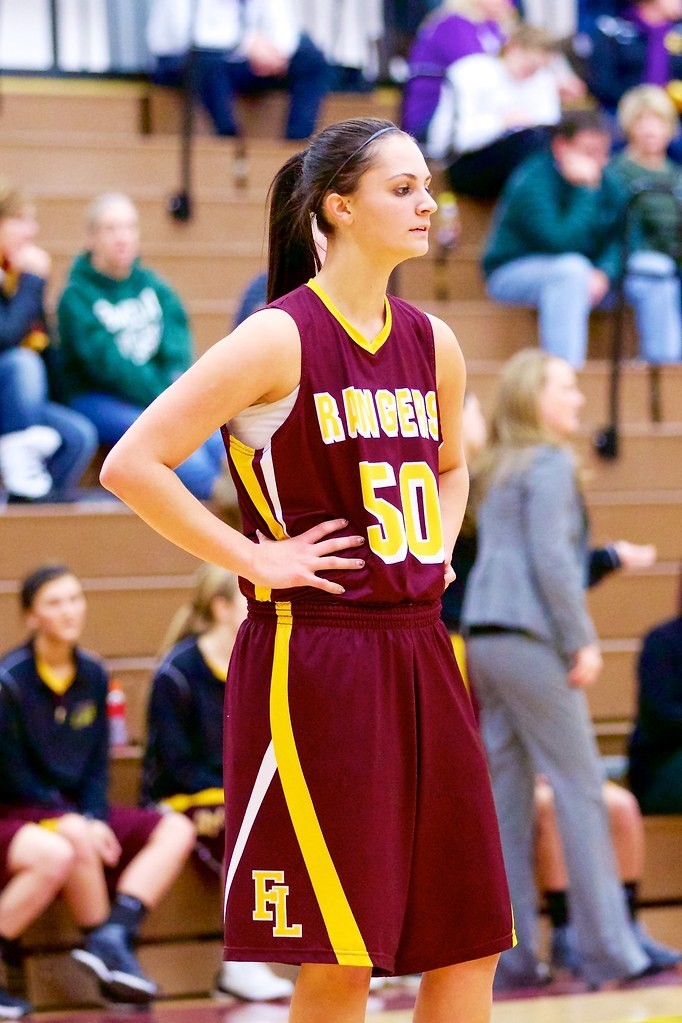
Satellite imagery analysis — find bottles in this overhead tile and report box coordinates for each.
[436,190,461,247]
[106,680,127,747]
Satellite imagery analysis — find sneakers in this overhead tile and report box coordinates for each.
[216,956,293,1000]
[0,988,30,1018]
[70,924,160,997]
[549,923,581,971]
[625,923,678,967]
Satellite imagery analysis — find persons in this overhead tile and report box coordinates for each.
[0,185,104,514]
[0,559,151,1017]
[0,801,155,1022]
[584,0,682,160]
[420,21,590,221]
[95,114,522,1023]
[427,383,649,996]
[136,556,392,1014]
[401,0,542,162]
[140,0,336,170]
[231,207,333,327]
[594,82,682,330]
[55,191,256,519]
[455,344,669,998]
[625,564,682,818]
[532,775,678,980]
[480,109,682,445]
